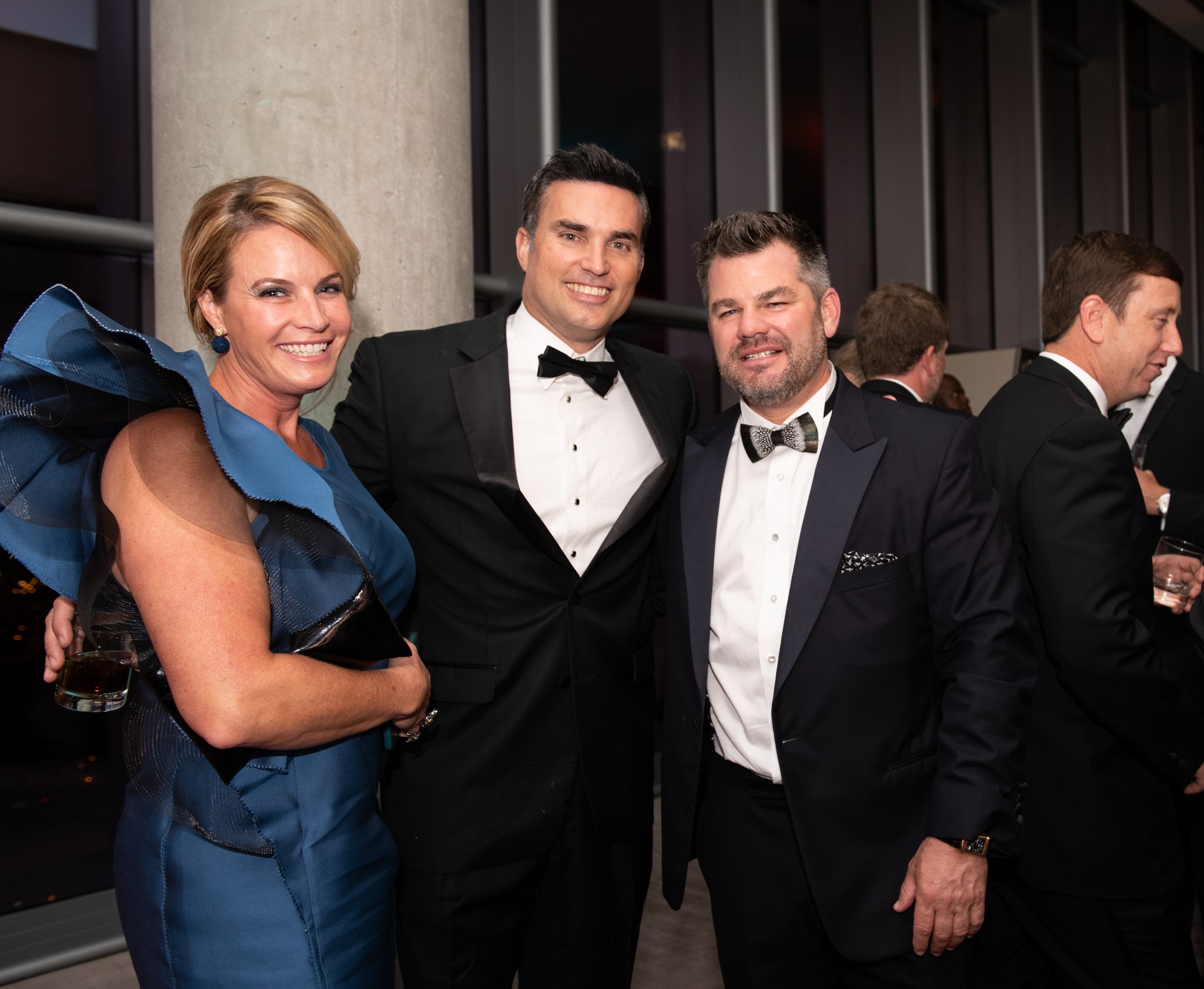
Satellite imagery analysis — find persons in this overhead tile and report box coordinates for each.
[658,207,1204,989]
[0,174,431,989]
[44,142,897,989]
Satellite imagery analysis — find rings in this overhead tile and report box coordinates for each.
[404,709,437,742]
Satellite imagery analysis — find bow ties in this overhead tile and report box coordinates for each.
[1106,407,1134,431]
[537,345,618,398]
[740,381,838,463]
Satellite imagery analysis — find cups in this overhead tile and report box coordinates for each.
[53,611,138,713]
[1152,537,1204,610]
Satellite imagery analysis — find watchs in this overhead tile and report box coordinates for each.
[936,832,991,858]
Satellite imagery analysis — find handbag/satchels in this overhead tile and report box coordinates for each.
[140,576,412,784]
